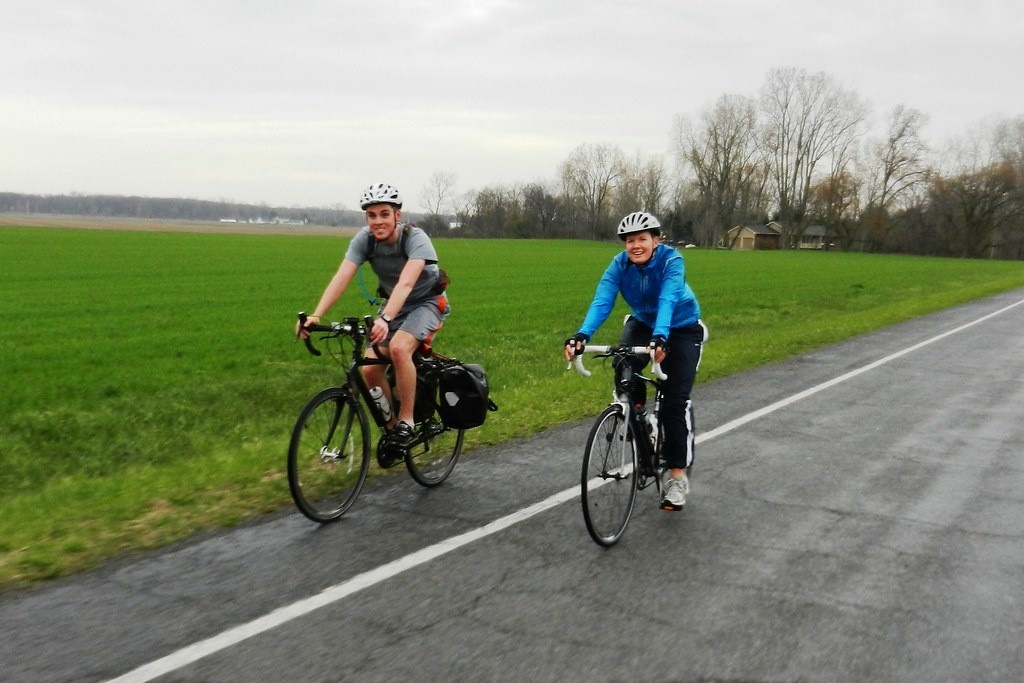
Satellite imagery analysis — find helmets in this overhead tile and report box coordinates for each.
[617,212,661,241]
[359,184,402,211]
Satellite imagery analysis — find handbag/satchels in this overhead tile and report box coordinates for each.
[387,356,436,423]
[439,362,488,429]
[402,268,447,307]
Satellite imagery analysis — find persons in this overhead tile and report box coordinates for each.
[565,212,709,504]
[295,184,450,445]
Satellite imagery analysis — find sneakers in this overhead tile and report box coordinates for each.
[620,416,653,442]
[660,474,689,506]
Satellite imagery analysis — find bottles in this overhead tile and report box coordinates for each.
[634,403,653,434]
[369,386,391,421]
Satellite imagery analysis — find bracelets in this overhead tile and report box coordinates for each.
[310,314,320,323]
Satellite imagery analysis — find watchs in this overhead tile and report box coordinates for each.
[379,313,392,325]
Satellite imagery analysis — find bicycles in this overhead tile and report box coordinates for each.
[287,311,466,525]
[565,339,665,549]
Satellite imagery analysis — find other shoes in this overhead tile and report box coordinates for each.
[389,420,415,442]
